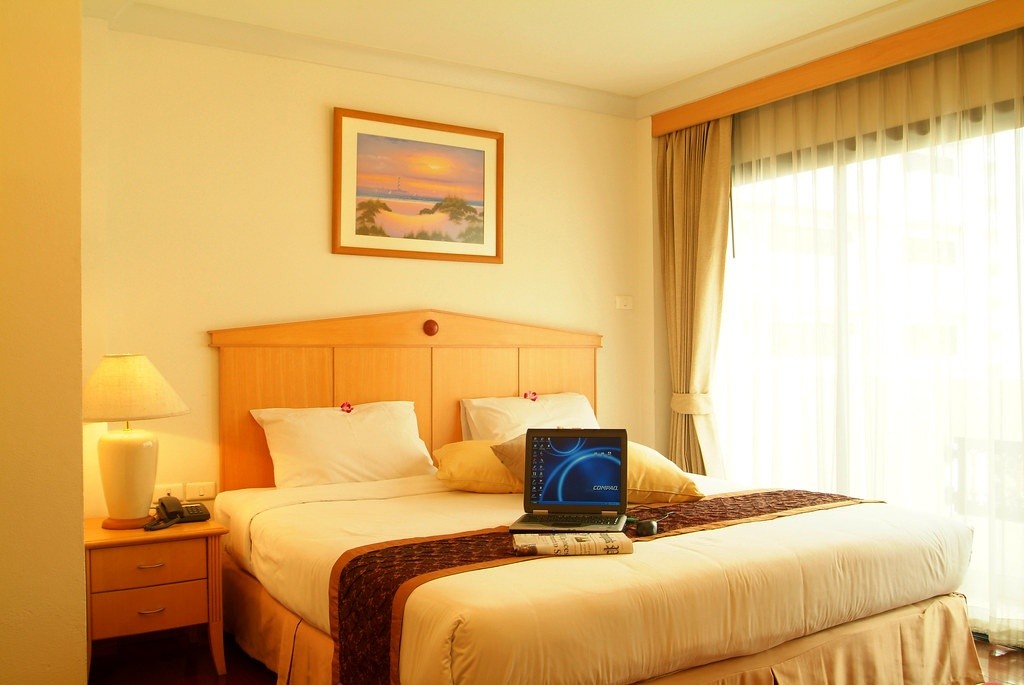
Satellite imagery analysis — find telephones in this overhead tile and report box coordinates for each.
[154,494,212,525]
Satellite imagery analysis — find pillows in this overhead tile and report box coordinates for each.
[489,433,706,505]
[459,392,600,439]
[432,440,523,494]
[251,400,438,489]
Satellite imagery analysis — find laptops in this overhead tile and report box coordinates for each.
[510,428,627,535]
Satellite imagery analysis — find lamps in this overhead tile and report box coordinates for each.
[81,352,192,531]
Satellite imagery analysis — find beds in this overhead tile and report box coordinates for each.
[206,307,986,685]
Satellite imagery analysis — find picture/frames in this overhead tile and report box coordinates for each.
[331,106,504,265]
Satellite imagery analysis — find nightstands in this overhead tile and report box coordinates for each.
[85,512,231,675]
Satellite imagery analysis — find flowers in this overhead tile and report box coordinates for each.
[523,390,538,401]
[341,402,353,414]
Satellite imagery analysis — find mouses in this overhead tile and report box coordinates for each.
[636,520,658,537]
